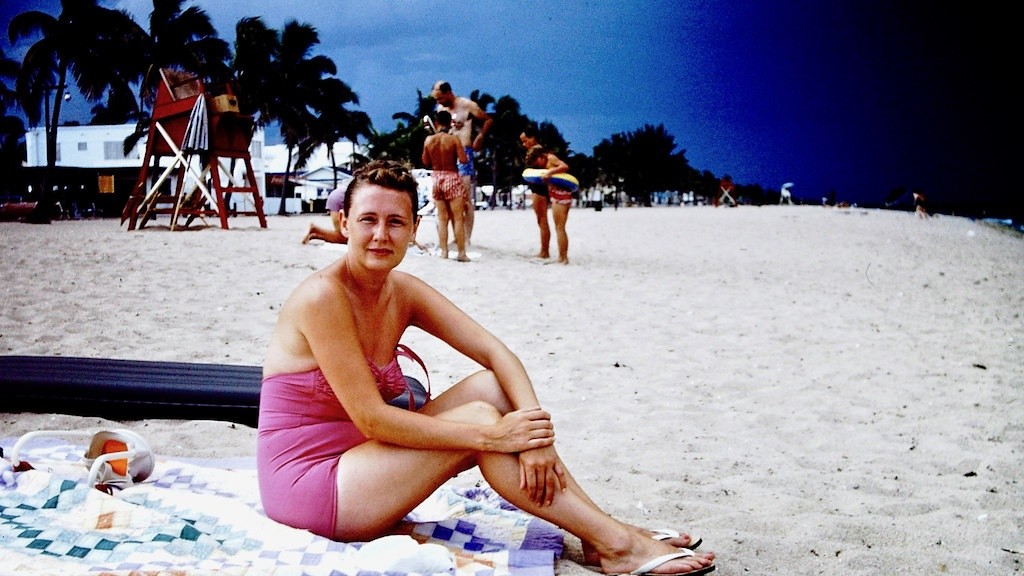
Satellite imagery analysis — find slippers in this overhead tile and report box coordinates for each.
[608,547,716,576]
[650,527,704,550]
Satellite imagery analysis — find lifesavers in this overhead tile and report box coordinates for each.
[521,168,580,193]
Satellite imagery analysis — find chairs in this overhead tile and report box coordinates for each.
[160,69,254,123]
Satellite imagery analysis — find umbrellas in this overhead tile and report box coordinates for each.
[170,93,208,232]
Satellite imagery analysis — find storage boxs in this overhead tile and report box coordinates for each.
[209,94,238,112]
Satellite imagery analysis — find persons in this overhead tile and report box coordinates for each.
[423,81,493,263]
[303,177,429,250]
[180,193,186,204]
[257,157,716,576]
[519,127,572,266]
[482,177,695,211]
[911,189,927,220]
[55,198,70,220]
